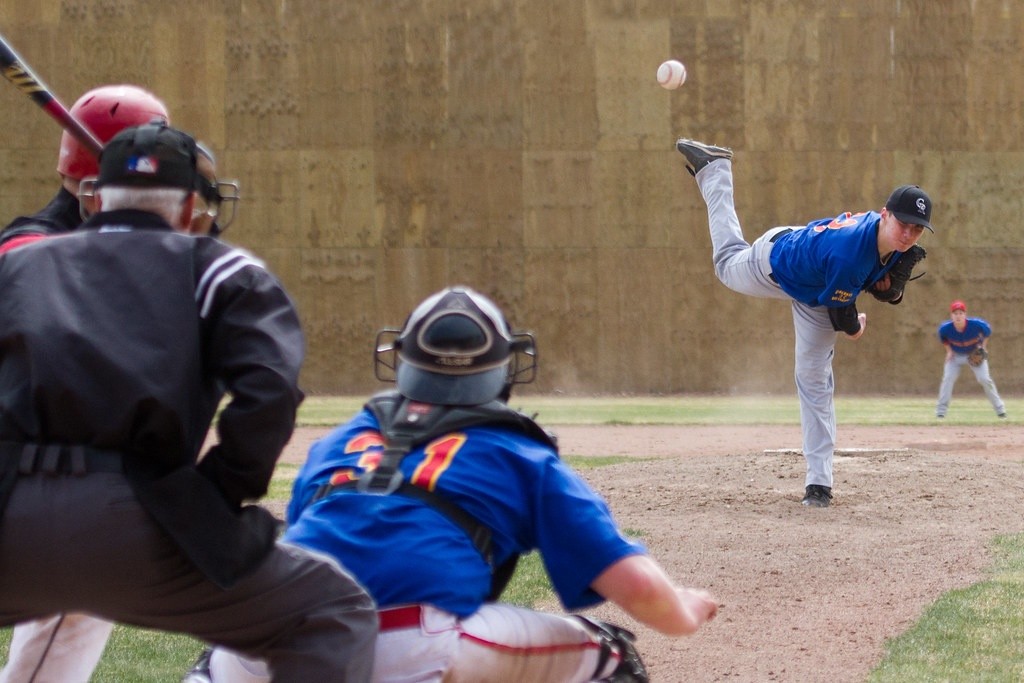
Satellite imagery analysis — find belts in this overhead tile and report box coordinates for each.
[766,228,793,284]
[17,447,133,477]
[373,605,422,632]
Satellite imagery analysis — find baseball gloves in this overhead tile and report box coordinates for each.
[967,347,987,366]
[865,243,927,302]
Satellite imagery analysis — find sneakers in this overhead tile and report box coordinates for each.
[803,484,833,507]
[676,139,734,176]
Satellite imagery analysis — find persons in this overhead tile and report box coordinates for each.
[1,86,378,683]
[676,139,934,506]
[936,302,1006,419]
[188,285,718,683]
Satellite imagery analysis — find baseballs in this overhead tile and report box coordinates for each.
[656,59,687,90]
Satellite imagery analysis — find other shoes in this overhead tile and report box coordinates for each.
[937,415,944,418]
[181,648,213,683]
[998,413,1008,420]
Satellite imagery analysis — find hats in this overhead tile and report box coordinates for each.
[952,302,966,312]
[887,185,935,233]
[94,121,198,190]
[394,286,511,405]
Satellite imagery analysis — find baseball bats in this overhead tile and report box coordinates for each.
[1,36,104,159]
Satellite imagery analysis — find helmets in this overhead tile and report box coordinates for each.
[55,84,171,181]
